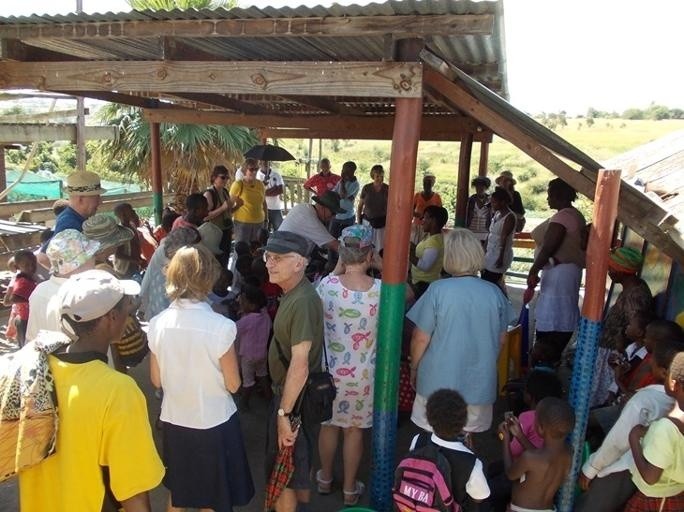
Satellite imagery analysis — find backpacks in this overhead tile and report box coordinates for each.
[392,433,462,512]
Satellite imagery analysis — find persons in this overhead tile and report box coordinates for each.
[0,161,684,512]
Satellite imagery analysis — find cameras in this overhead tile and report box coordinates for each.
[224,217,232,227]
[608,350,626,369]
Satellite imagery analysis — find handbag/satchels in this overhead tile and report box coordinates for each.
[532,218,582,263]
[370,216,386,228]
[301,372,336,424]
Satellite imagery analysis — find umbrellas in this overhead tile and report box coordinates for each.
[243,145,296,176]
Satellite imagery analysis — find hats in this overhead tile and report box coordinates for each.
[312,190,347,213]
[47,212,141,322]
[495,171,516,184]
[608,247,643,273]
[257,231,307,256]
[164,222,225,261]
[61,171,106,196]
[341,225,376,248]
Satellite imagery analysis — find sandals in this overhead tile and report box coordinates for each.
[316,470,333,494]
[343,481,365,506]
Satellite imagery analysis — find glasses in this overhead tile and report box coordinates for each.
[249,168,258,172]
[218,176,230,179]
[263,252,294,262]
